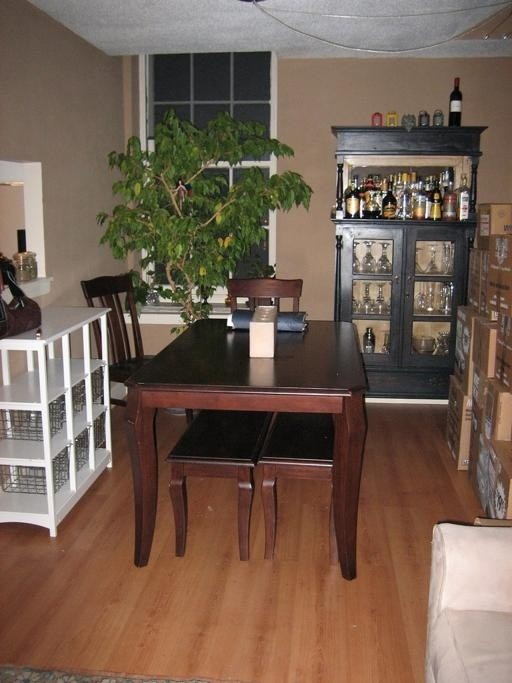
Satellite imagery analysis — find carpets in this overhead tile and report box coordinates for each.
[0,661,212,683]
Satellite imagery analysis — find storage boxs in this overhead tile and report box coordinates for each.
[447,201,512,518]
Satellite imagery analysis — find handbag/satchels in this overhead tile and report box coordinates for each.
[0,270,41,340]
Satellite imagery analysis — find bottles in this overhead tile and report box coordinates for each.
[426,287,435,312]
[353,241,360,271]
[425,243,439,274]
[378,241,392,273]
[447,76,464,127]
[374,282,388,313]
[362,327,376,354]
[362,240,377,273]
[344,168,470,224]
[441,242,454,274]
[359,282,374,314]
[14,251,37,282]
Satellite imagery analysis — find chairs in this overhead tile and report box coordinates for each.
[80,272,194,427]
[226,275,304,312]
[425,522,512,682]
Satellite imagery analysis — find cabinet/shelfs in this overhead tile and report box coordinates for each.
[329,124,489,403]
[0,306,115,536]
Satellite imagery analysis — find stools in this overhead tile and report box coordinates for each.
[168,411,267,560]
[256,410,331,566]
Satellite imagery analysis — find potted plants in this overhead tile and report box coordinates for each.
[96,107,313,415]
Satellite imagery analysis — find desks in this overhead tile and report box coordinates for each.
[123,317,369,579]
